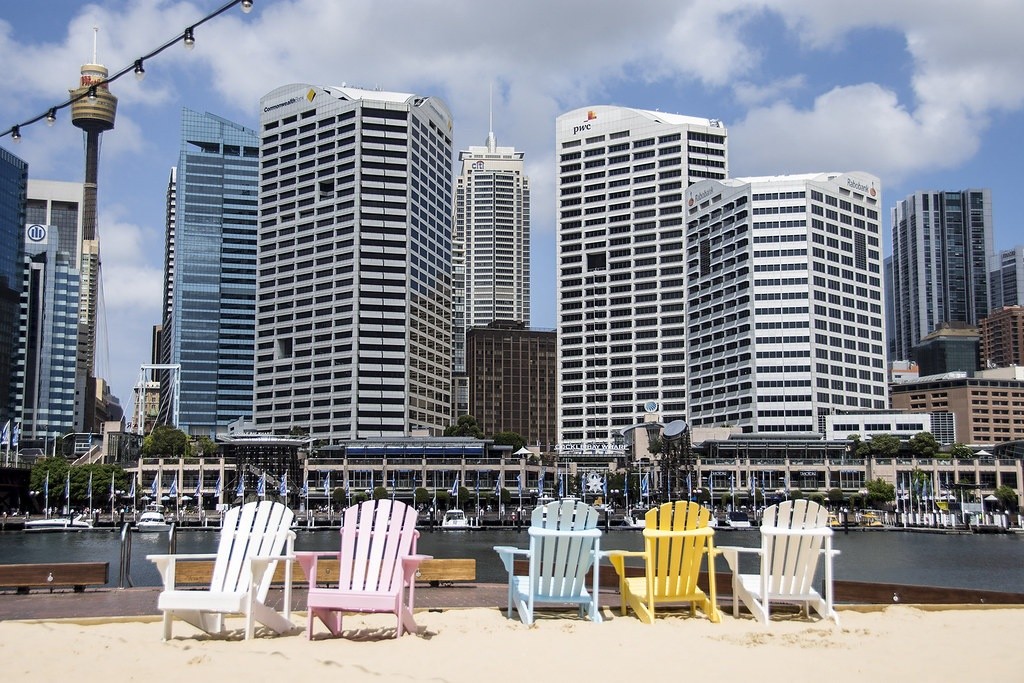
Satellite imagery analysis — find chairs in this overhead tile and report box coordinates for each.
[716,499,840,626]
[609,501,724,626]
[145,500,296,641]
[493,500,606,630]
[295,498,433,641]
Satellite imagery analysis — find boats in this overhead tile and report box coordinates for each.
[623,506,753,531]
[440,507,472,532]
[135,512,171,532]
[22,514,89,532]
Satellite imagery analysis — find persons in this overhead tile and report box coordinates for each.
[316,504,441,520]
[128,503,227,519]
[480,498,762,523]
[2,504,125,519]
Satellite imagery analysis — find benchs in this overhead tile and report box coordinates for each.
[512,560,761,600]
[175,560,476,590]
[0,562,110,595]
[820,579,1024,604]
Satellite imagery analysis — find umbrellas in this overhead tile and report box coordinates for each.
[139,495,193,504]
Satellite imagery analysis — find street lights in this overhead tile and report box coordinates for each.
[857,487,869,514]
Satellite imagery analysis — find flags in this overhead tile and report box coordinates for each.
[129,473,159,497]
[367,475,416,497]
[169,476,201,497]
[557,474,607,496]
[707,475,734,495]
[517,474,544,497]
[303,476,349,496]
[622,475,691,496]
[84,478,113,501]
[894,478,934,498]
[214,475,264,497]
[450,478,501,496]
[280,473,286,497]
[750,475,787,495]
[43,479,69,499]
